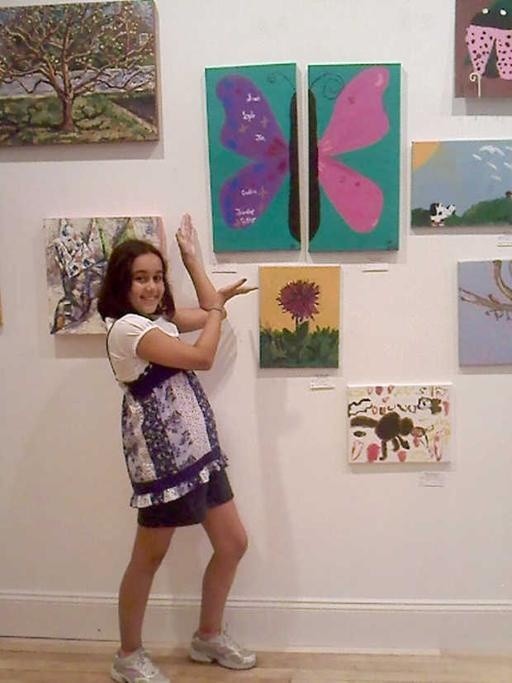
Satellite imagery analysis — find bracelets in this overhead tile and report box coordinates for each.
[208,304,227,319]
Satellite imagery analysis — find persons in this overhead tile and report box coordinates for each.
[98,213,259,683]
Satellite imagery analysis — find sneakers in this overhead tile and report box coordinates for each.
[111,645,170,683]
[188,631,256,669]
